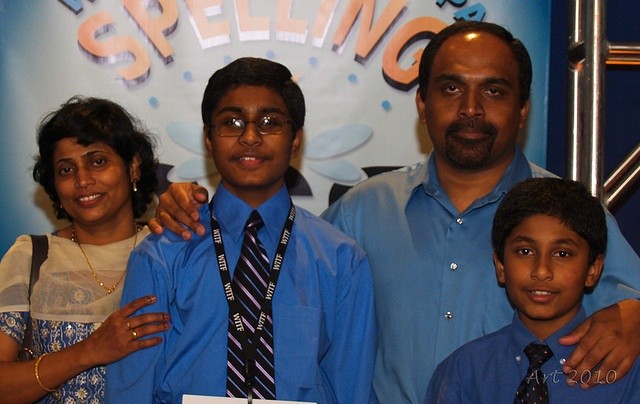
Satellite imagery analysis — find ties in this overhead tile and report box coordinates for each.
[226,210,276,400]
[513,344,552,403]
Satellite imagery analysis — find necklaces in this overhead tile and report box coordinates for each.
[65,220,143,295]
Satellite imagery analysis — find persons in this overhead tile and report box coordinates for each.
[0,96,208,404]
[425,177,639,401]
[107,56,377,403]
[319,21,637,403]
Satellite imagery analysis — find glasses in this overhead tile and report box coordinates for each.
[207,114,297,136]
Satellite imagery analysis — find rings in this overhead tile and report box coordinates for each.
[126,320,131,328]
[132,331,137,337]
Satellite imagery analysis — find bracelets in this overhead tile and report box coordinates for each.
[35,352,61,392]
